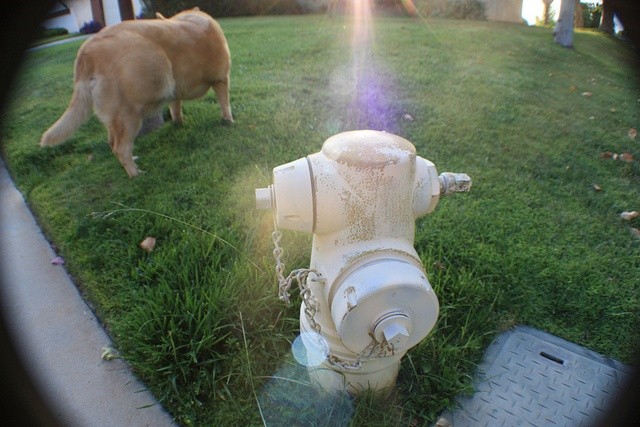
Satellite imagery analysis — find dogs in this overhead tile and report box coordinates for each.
[40,6,234,177]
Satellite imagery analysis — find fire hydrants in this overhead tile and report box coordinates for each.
[255,128,472,394]
